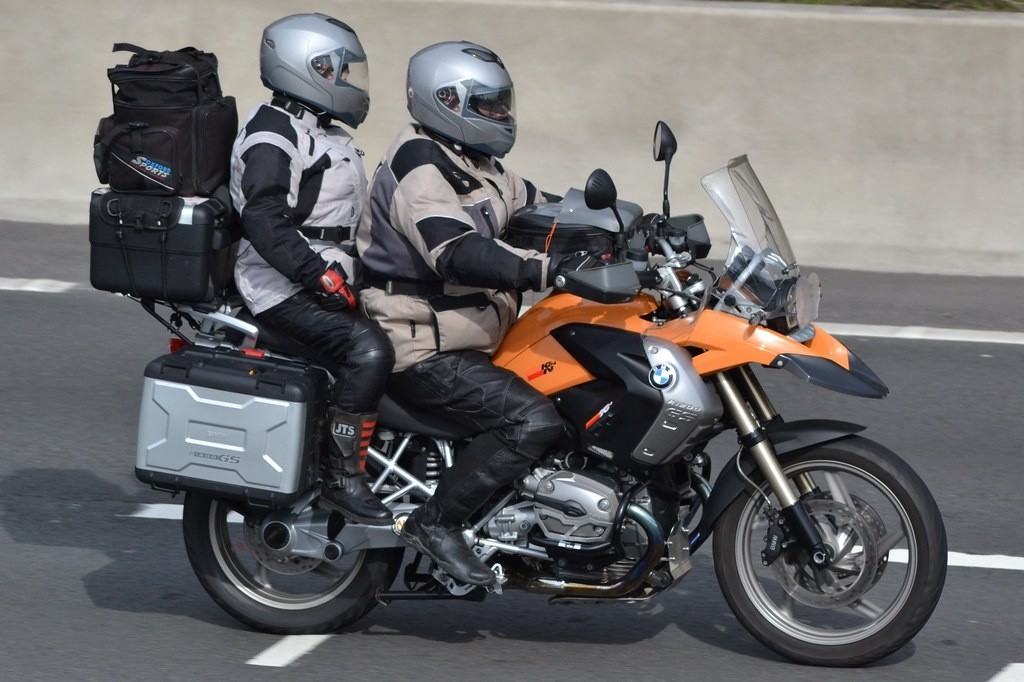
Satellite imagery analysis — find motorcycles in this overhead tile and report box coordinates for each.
[133,119,949,669]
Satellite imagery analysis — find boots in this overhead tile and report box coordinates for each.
[401,494,492,586]
[319,405,394,526]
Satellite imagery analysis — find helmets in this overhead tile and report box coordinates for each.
[406,41,517,158]
[260,13,370,129]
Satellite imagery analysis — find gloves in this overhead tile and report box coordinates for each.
[546,250,615,290]
[640,213,661,224]
[313,261,358,313]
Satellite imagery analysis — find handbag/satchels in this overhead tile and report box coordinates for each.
[94,42,238,197]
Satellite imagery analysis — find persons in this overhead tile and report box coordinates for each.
[230,13,395,526]
[355,40,684,586]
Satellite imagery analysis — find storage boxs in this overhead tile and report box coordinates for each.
[89,188,242,303]
[132,346,318,515]
